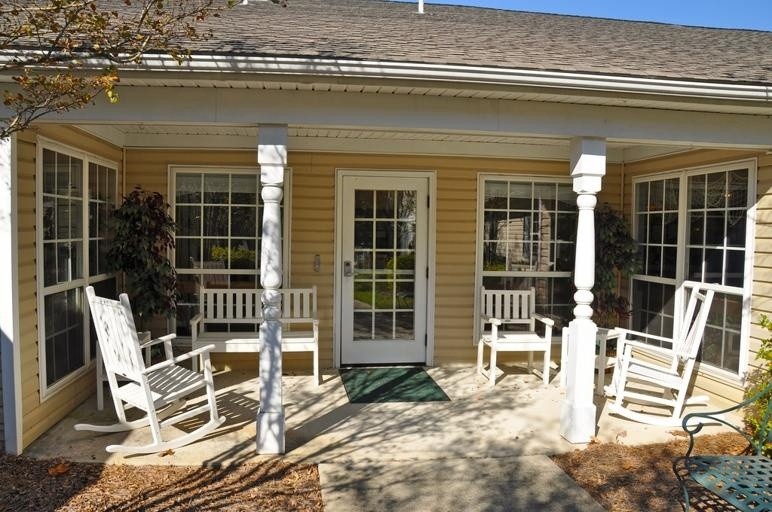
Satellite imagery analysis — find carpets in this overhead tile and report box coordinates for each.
[338,367,452,403]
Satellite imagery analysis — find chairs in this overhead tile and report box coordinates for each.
[189,257,227,285]
[73,285,227,456]
[602,281,728,428]
[477,286,555,389]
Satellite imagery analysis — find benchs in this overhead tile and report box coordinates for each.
[673,385,771,512]
[188,283,323,387]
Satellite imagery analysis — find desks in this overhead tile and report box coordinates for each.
[90,332,154,415]
[559,326,623,402]
